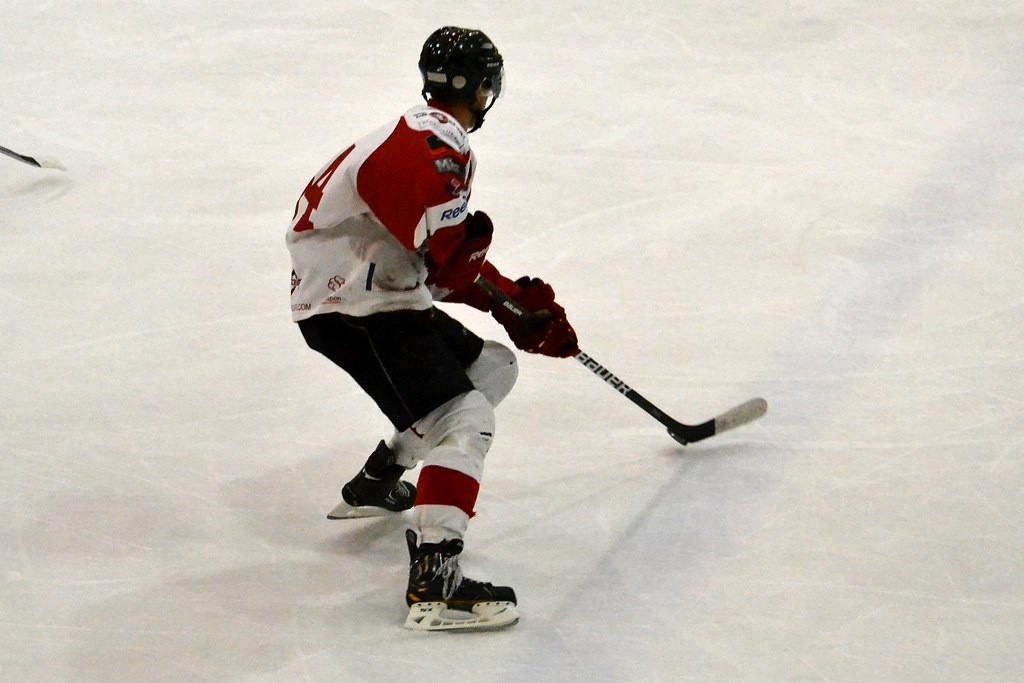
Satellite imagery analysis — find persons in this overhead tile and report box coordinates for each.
[285,25,580,631]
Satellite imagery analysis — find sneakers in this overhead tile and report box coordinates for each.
[326,440,417,520]
[402,528,519,631]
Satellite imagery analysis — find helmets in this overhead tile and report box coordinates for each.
[418,25,503,99]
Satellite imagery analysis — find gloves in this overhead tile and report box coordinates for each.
[424,211,495,313]
[492,277,578,358]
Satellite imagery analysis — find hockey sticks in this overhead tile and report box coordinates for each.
[472,272,769,447]
[0,146,68,171]
